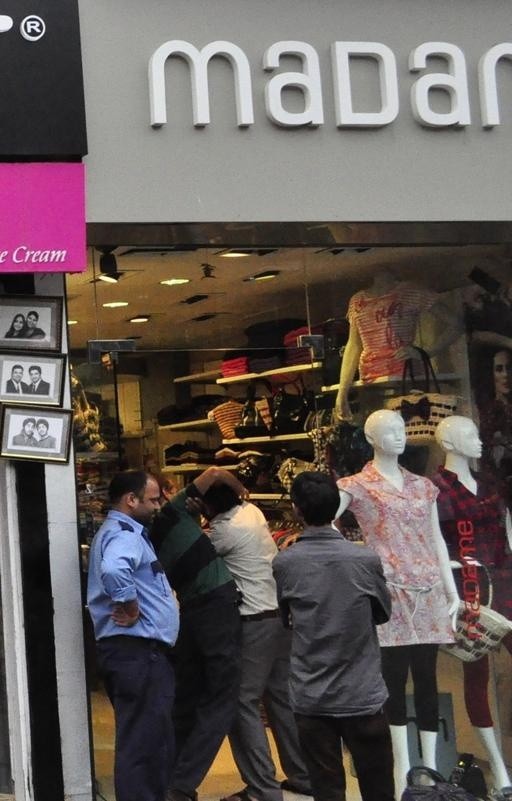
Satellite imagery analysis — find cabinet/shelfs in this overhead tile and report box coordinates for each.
[158,313,512,500]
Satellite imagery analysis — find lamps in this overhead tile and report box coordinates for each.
[93,243,122,283]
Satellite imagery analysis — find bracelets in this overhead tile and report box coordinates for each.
[445,590,457,595]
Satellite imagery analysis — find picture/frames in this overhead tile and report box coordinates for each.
[0,296,63,353]
[0,403,74,465]
[0,353,66,407]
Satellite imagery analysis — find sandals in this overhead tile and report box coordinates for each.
[220,791,261,801]
[280,780,313,795]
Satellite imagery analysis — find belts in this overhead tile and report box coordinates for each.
[240,610,281,622]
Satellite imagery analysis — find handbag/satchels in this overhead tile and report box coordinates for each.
[402,766,479,801]
[382,392,458,446]
[73,409,105,452]
[439,599,512,664]
[213,391,370,494]
[323,333,359,386]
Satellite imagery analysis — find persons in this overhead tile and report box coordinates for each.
[330,408,460,801]
[198,481,315,801]
[87,468,181,800]
[426,414,511,801]
[147,466,250,800]
[5,364,50,395]
[12,417,56,448]
[4,311,46,340]
[326,266,467,419]
[272,471,397,801]
